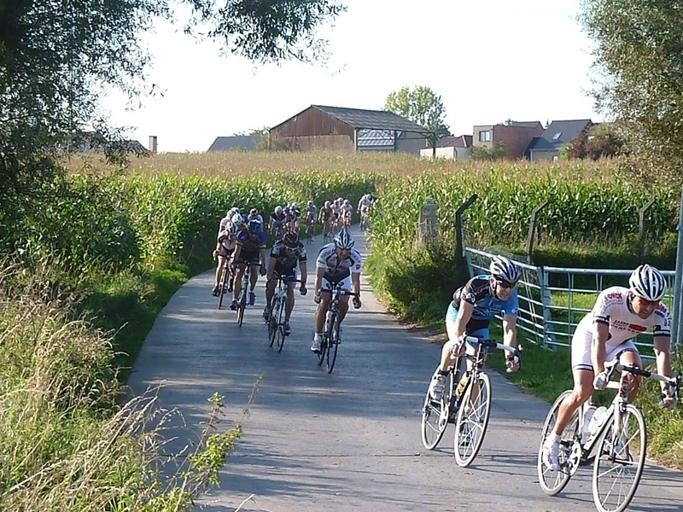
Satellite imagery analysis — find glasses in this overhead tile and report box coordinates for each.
[287,248,298,252]
[638,298,659,308]
[498,282,516,288]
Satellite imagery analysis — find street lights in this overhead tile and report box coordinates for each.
[261,130,270,152]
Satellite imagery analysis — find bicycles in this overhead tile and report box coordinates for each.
[533,356,682,511]
[211,252,233,310]
[229,257,262,329]
[266,272,305,354]
[419,332,528,469]
[312,281,360,375]
[266,212,370,246]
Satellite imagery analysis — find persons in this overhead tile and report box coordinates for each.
[540,264,676,471]
[211,193,375,311]
[263,230,305,335]
[427,253,520,447]
[309,226,361,354]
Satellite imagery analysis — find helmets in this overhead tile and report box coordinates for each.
[274,202,297,213]
[225,207,263,235]
[307,201,313,207]
[324,197,350,207]
[334,227,355,250]
[283,232,300,247]
[362,194,372,204]
[490,254,523,284]
[630,264,668,301]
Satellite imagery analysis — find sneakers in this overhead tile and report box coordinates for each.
[310,334,323,352]
[333,328,343,343]
[248,292,256,306]
[429,365,448,400]
[262,307,273,321]
[213,285,218,293]
[542,436,561,471]
[284,323,292,334]
[229,301,237,309]
